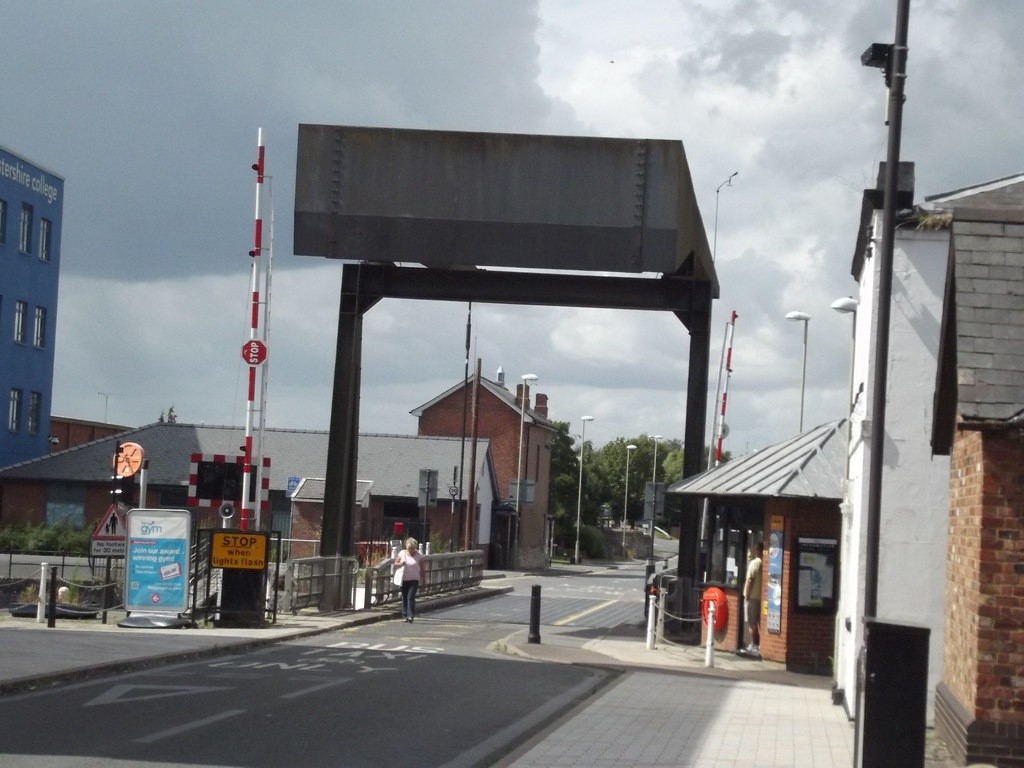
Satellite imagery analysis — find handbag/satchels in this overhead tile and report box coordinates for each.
[394,550,406,587]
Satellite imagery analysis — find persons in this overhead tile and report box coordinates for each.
[393,537,426,623]
[737,543,764,655]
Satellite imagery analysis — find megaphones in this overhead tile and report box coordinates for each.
[219,503,234,518]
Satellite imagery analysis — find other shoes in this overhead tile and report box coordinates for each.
[402,618,407,621]
[409,617,413,623]
[740,644,759,657]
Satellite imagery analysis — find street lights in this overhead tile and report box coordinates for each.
[622,444,639,558]
[513,373,538,563]
[575,414,594,566]
[785,311,812,435]
[832,296,860,524]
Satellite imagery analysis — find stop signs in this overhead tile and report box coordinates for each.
[241,339,271,369]
